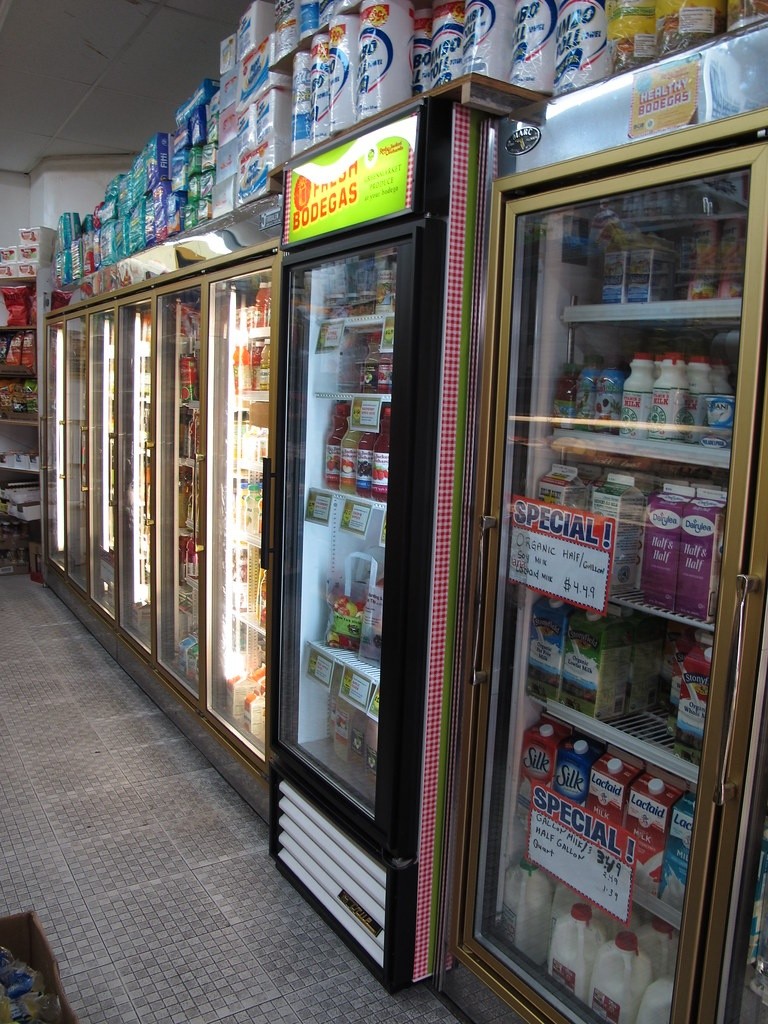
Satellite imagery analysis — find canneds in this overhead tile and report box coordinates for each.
[181,353,198,403]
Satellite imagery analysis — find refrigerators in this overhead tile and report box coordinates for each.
[44,16,768,1024]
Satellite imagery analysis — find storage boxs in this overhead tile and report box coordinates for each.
[0,453,44,584]
[0,910,79,1024]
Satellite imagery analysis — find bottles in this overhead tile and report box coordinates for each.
[225,279,270,744]
[370,406,391,500]
[590,932,651,1024]
[359,335,392,393]
[621,352,731,444]
[551,363,577,429]
[578,356,598,429]
[502,858,550,965]
[335,695,378,783]
[548,903,604,999]
[176,353,201,580]
[597,355,626,432]
[356,433,378,496]
[323,404,350,490]
[338,418,363,494]
[634,978,673,1024]
[632,919,678,977]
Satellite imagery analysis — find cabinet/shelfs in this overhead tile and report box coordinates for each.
[0,275,45,523]
